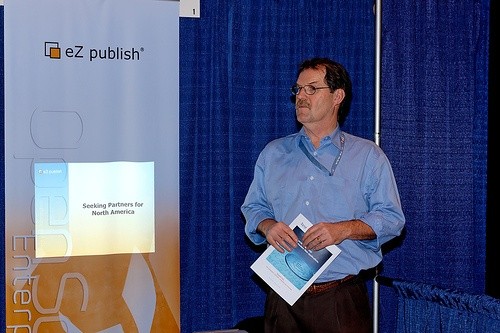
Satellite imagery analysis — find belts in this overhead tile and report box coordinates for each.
[306,275,355,292]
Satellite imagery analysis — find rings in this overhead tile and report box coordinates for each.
[316,237,322,242]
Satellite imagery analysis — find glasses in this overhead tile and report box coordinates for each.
[290,85,330,95]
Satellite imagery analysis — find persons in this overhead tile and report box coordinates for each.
[241,57,407,332]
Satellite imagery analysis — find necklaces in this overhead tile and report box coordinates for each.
[298,133,345,176]
